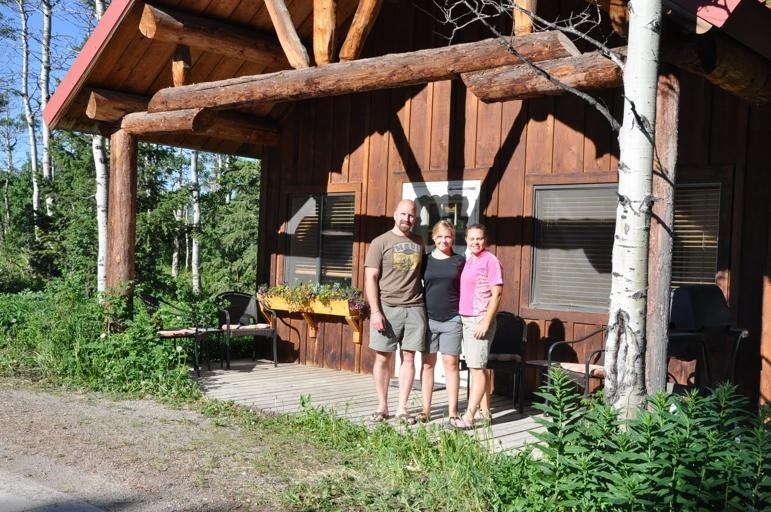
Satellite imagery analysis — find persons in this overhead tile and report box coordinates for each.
[364,199,426,426]
[453,223,506,430]
[408,219,464,428]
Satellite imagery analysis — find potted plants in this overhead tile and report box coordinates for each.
[257,282,363,342]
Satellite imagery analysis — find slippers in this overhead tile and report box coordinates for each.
[449,412,493,430]
[396,411,432,427]
[369,411,388,423]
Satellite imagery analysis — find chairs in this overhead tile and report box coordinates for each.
[136,292,220,376]
[547,328,605,397]
[213,291,277,372]
[460,312,529,408]
[667,285,750,392]
